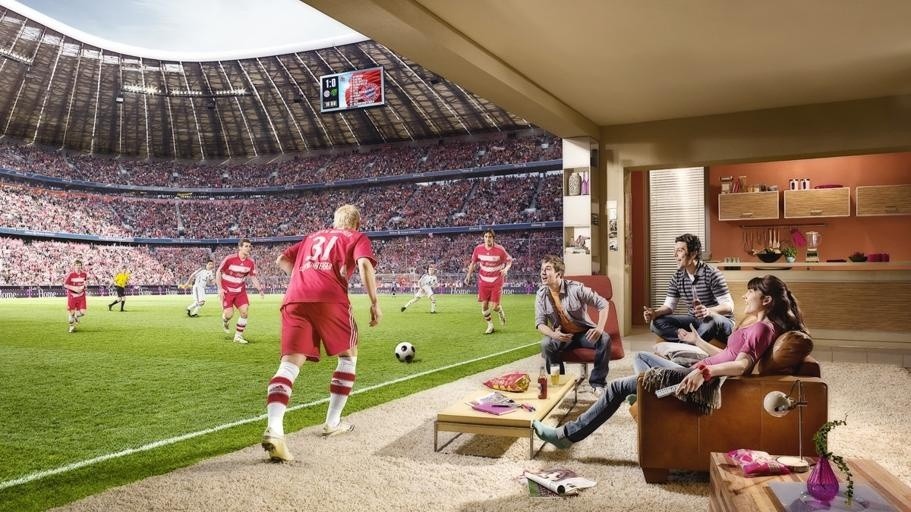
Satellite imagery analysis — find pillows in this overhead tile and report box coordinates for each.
[749,328,814,377]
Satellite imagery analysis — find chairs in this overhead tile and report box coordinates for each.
[540,275,624,378]
[630,335,826,482]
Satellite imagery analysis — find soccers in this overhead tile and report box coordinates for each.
[394,341,416,361]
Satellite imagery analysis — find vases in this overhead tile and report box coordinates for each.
[568,173,581,195]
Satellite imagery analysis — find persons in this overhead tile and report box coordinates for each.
[534,254,612,398]
[0,131,563,297]
[530,275,814,449]
[185,260,217,318]
[402,268,439,313]
[644,234,734,345]
[261,205,381,464]
[108,266,130,311]
[216,238,259,343]
[464,230,512,334]
[64,260,87,333]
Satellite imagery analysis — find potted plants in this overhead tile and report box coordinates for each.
[804,416,855,506]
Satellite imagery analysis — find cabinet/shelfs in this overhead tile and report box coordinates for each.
[784,186,850,219]
[718,191,779,221]
[855,183,911,217]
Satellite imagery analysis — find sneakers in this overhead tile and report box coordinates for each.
[485,328,495,334]
[321,422,357,438]
[499,315,506,326]
[222,323,230,333]
[431,311,437,313]
[108,304,112,311]
[120,309,127,312]
[262,429,295,463]
[233,335,248,344]
[401,306,406,312]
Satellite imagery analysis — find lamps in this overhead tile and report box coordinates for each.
[762,380,812,473]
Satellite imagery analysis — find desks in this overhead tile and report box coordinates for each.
[708,451,911,512]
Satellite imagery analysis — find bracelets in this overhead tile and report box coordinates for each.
[696,364,711,381]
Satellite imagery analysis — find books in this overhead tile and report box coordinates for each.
[472,402,516,415]
[526,466,598,498]
